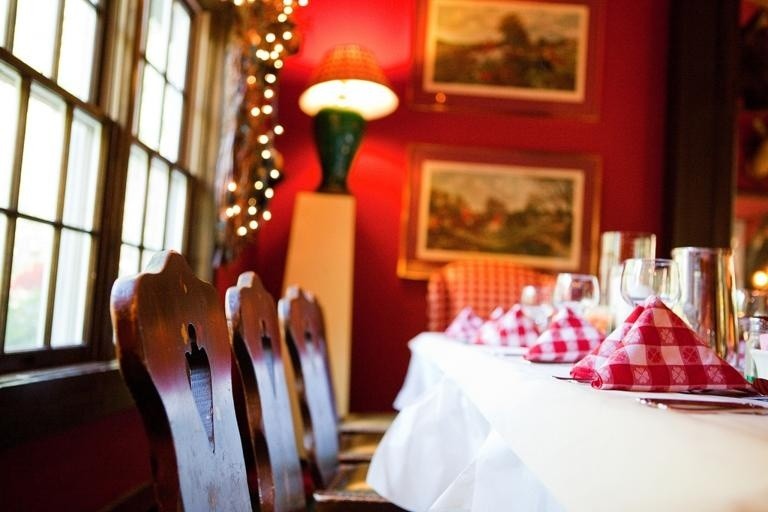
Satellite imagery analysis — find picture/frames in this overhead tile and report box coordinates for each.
[396,141,606,291]
[409,1,600,123]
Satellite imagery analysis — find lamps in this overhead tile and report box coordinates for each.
[299,44,401,194]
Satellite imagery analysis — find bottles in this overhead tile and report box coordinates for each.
[745,315,768,396]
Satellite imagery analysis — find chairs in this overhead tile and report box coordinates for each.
[106,249,409,512]
[425,259,555,336]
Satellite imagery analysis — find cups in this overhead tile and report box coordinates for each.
[517,229,683,318]
[671,245,741,367]
